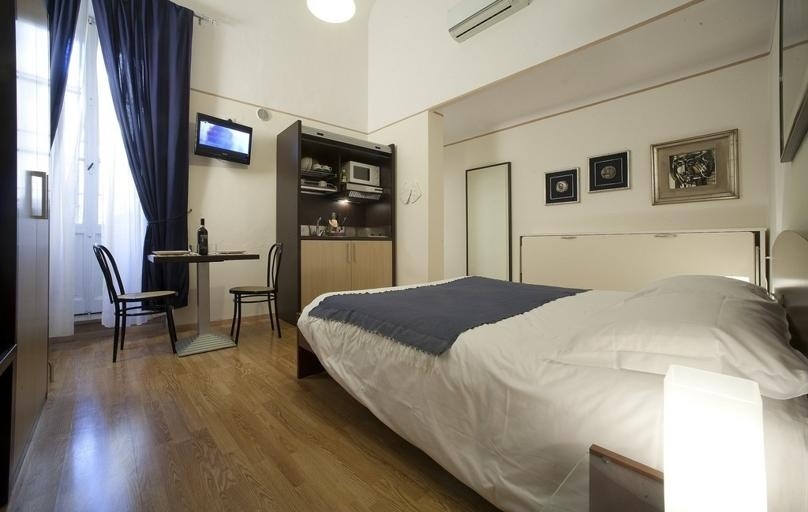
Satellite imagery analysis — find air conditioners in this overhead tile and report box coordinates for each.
[446,0,530,44]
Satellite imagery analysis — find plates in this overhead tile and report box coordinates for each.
[214,249,247,254]
[302,155,312,173]
[151,250,190,255]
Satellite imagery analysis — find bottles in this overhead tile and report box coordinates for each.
[197,218,209,256]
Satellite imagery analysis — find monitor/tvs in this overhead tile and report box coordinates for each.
[194,112,253,165]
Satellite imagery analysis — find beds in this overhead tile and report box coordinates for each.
[296,275,808,512]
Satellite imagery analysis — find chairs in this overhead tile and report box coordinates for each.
[93,244,179,364]
[230,243,284,346]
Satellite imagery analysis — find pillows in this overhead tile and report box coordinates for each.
[537,274,808,401]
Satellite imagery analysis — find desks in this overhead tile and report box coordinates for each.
[147,251,260,359]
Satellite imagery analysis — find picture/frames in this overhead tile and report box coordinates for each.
[778,0,808,163]
[543,167,581,206]
[650,128,740,206]
[586,150,631,193]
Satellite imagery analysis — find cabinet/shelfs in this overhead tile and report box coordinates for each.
[275,120,397,327]
[0,2,50,448]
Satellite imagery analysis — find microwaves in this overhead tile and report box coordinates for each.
[344,160,380,187]
